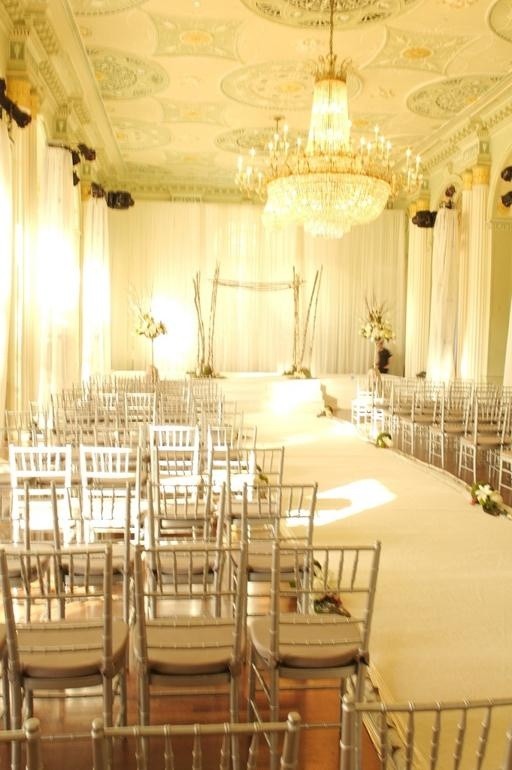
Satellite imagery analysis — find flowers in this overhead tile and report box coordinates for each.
[360,287,396,344]
[131,282,166,341]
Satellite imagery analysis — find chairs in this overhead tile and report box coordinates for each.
[335,687,512,770]
[3,371,385,770]
[350,375,512,512]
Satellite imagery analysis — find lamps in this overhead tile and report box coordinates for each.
[234,0,421,239]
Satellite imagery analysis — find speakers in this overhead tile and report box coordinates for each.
[412,211,438,227]
[107,191,134,209]
